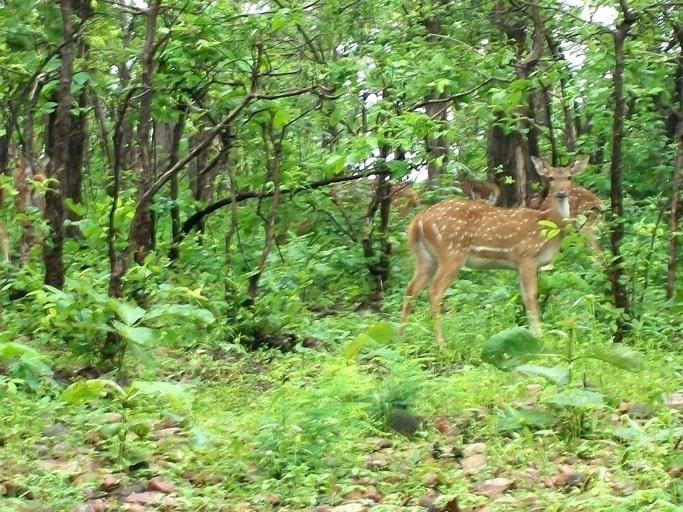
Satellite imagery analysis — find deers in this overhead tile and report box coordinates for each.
[276,178,604,257]
[0,134,47,280]
[396,153,588,350]
